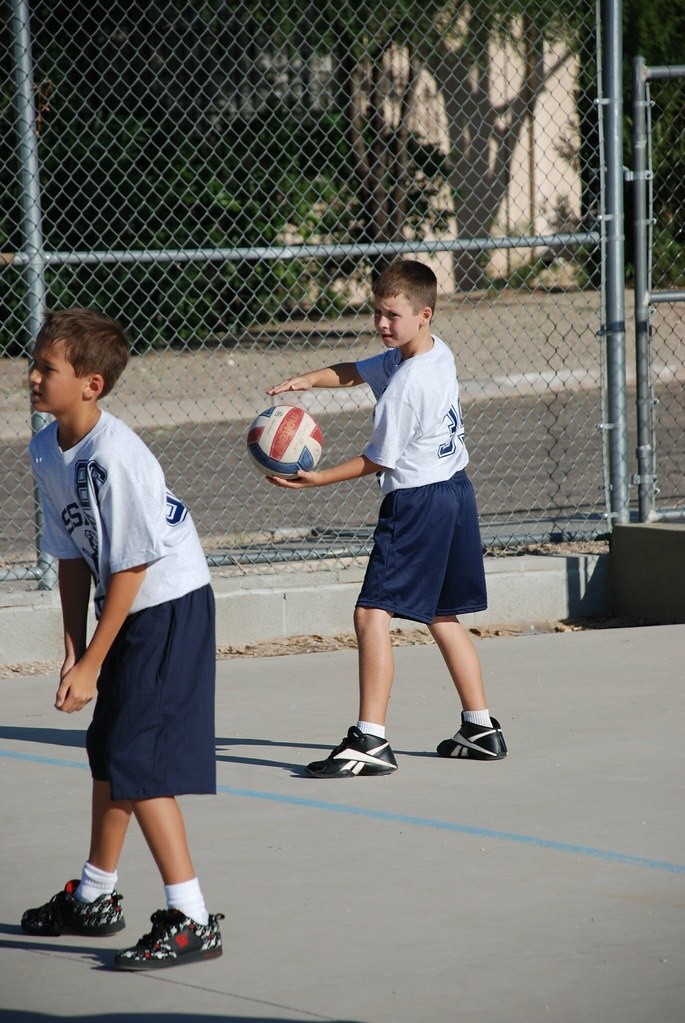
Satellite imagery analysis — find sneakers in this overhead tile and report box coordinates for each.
[116,908,225,972]
[305,726,398,778]
[437,711,507,760]
[20,880,126,937]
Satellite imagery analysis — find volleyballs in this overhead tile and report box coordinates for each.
[247,404,326,480]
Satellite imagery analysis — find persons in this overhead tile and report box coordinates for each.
[20,308,224,971]
[263,259,506,777]
